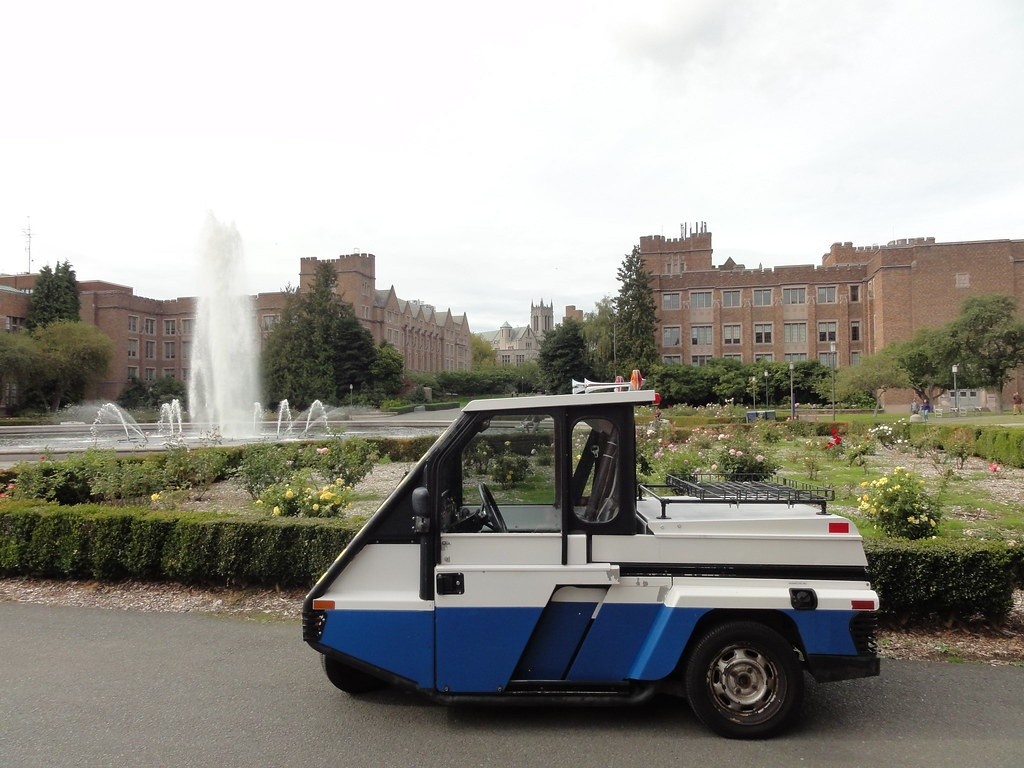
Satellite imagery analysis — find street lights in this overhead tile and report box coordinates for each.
[764,369,769,411]
[521,376,524,393]
[349,383,353,421]
[788,362,795,420]
[751,375,756,411]
[951,364,958,408]
[829,341,838,421]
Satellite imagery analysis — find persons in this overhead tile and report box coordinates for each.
[911,398,920,414]
[1012,392,1023,415]
[921,399,930,421]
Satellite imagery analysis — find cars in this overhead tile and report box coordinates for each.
[299,371,884,740]
